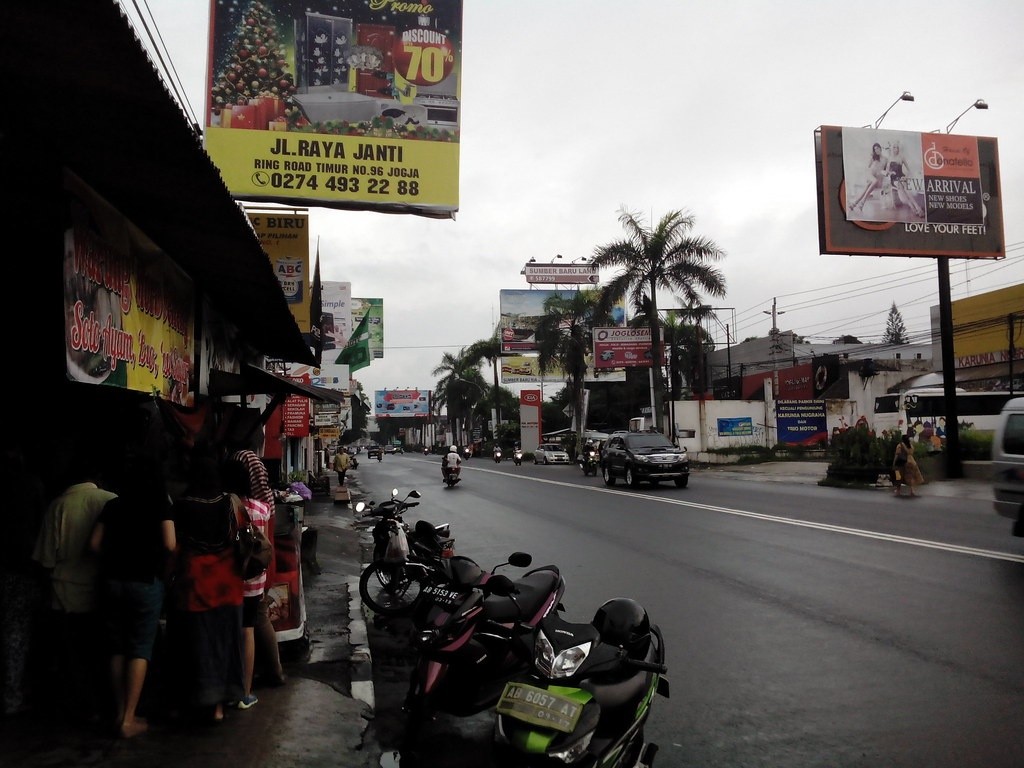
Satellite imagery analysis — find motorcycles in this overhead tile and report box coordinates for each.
[513,447,523,465]
[349,486,567,768]
[462,450,471,460]
[493,450,502,463]
[440,456,462,487]
[491,595,672,768]
[578,450,598,476]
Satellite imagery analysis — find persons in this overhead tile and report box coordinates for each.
[582,440,595,462]
[849,143,888,211]
[0,450,285,738]
[512,445,520,452]
[893,435,924,496]
[324,445,330,469]
[441,446,461,483]
[463,446,470,453]
[886,144,924,217]
[493,444,502,451]
[333,447,350,485]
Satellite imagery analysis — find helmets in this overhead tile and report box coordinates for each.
[591,598,651,661]
[450,445,457,452]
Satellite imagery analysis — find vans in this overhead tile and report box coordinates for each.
[978,395,1024,538]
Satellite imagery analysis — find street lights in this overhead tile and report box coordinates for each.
[762,310,786,370]
[459,378,484,399]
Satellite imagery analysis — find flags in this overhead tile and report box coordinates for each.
[335,310,370,372]
[310,248,324,352]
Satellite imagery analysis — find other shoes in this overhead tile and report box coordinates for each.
[122,718,148,738]
[276,671,288,685]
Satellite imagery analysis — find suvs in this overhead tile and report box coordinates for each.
[598,429,690,492]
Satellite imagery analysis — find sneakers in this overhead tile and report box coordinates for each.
[238,695,259,709]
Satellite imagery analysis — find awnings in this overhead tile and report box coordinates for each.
[244,363,345,440]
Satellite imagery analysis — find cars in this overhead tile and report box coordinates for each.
[367,445,380,459]
[384,445,395,455]
[532,443,570,466]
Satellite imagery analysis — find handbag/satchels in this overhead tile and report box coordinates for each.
[384,519,410,564]
[896,443,907,467]
[230,493,273,581]
